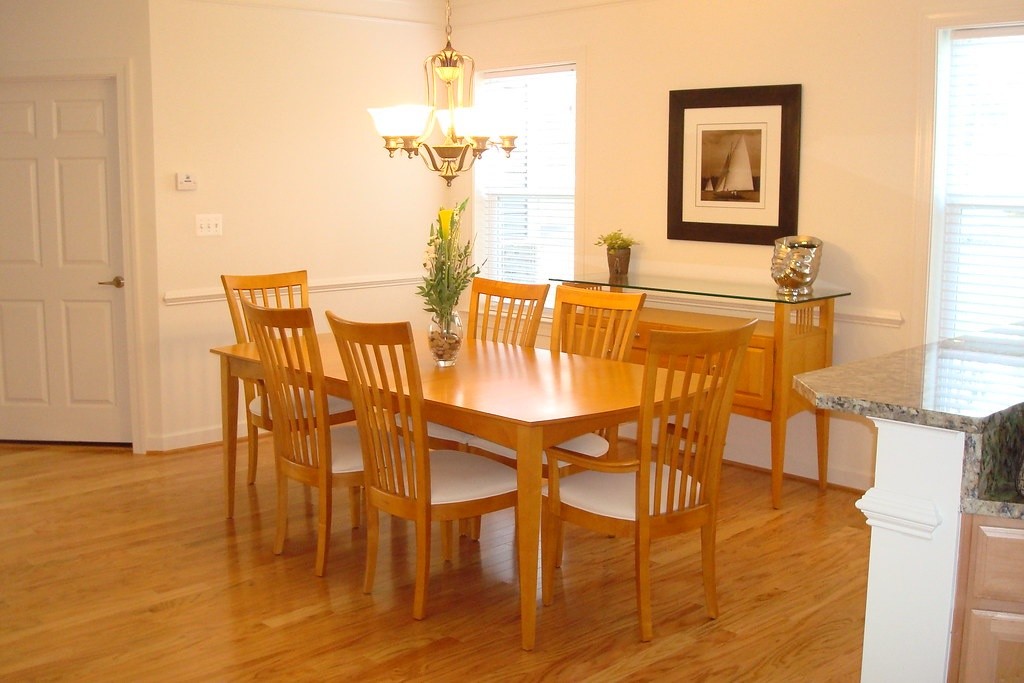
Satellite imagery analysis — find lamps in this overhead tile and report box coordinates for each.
[367,0,526,187]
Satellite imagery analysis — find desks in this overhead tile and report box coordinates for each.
[209,328,725,651]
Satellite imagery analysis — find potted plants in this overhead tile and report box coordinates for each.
[595,228,640,276]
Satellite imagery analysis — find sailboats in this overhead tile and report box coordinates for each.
[712,133,755,200]
[704,178,714,192]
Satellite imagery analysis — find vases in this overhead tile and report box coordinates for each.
[428,309,463,367]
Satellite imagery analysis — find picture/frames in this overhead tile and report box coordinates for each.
[666,83,802,246]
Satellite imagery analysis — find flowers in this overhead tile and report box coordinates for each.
[414,197,487,336]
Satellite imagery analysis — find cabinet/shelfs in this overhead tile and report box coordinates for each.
[548,273,853,509]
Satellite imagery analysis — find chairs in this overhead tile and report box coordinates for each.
[394,276,551,538]
[220,269,355,486]
[241,294,416,576]
[325,310,520,619]
[544,317,761,642]
[470,283,646,569]
